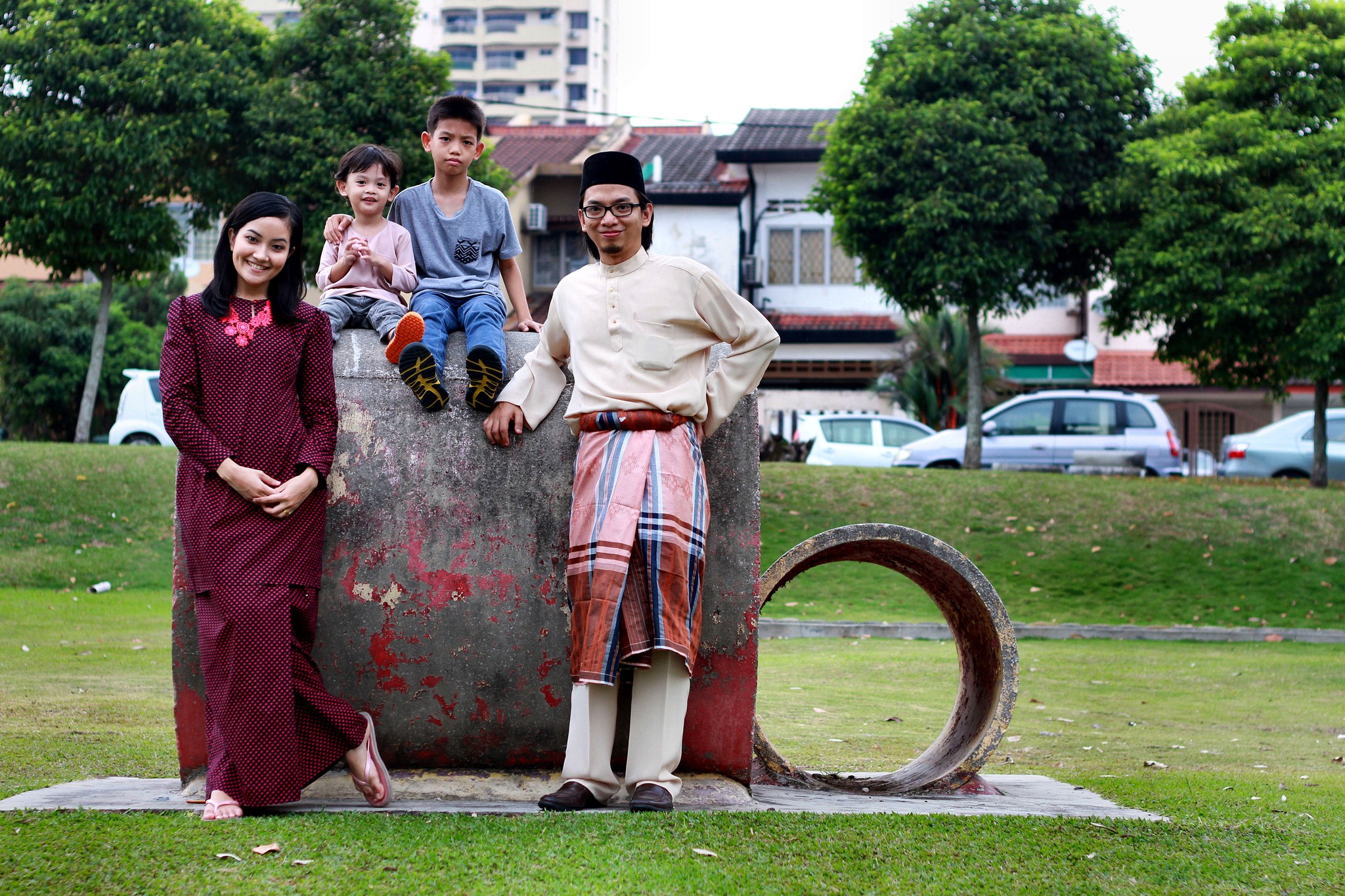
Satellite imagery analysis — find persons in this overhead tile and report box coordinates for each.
[484,152,780,813]
[158,191,394,823]
[316,143,426,364]
[322,95,543,414]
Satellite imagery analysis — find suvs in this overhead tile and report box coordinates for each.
[890,385,1187,480]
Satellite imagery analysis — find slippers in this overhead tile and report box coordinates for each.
[202,790,243,819]
[350,711,393,808]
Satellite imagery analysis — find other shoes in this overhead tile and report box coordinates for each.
[399,342,449,413]
[385,311,425,364]
[465,345,504,413]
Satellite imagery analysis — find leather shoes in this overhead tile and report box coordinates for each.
[630,783,674,811]
[538,782,609,811]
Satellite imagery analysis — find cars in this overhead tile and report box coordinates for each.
[1218,407,1345,484]
[108,368,176,448]
[789,412,937,470]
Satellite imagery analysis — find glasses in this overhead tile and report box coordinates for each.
[581,203,645,219]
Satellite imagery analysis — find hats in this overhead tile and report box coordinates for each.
[581,151,646,196]
